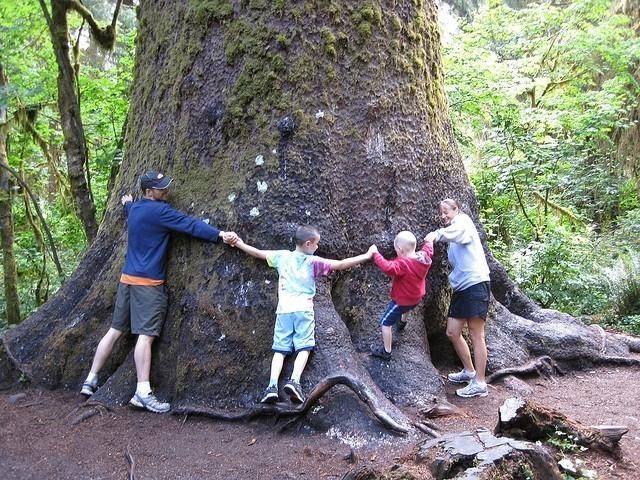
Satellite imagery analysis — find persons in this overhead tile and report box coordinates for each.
[422,198,492,398]
[79,169,246,415]
[371,230,435,362]
[221,224,378,407]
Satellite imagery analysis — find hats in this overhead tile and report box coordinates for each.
[141,171,175,190]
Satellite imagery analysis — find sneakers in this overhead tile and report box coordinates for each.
[370,344,391,361]
[447,369,477,384]
[397,314,406,330]
[455,379,488,398]
[129,390,171,413]
[80,381,101,396]
[284,379,305,404]
[260,385,278,404]
